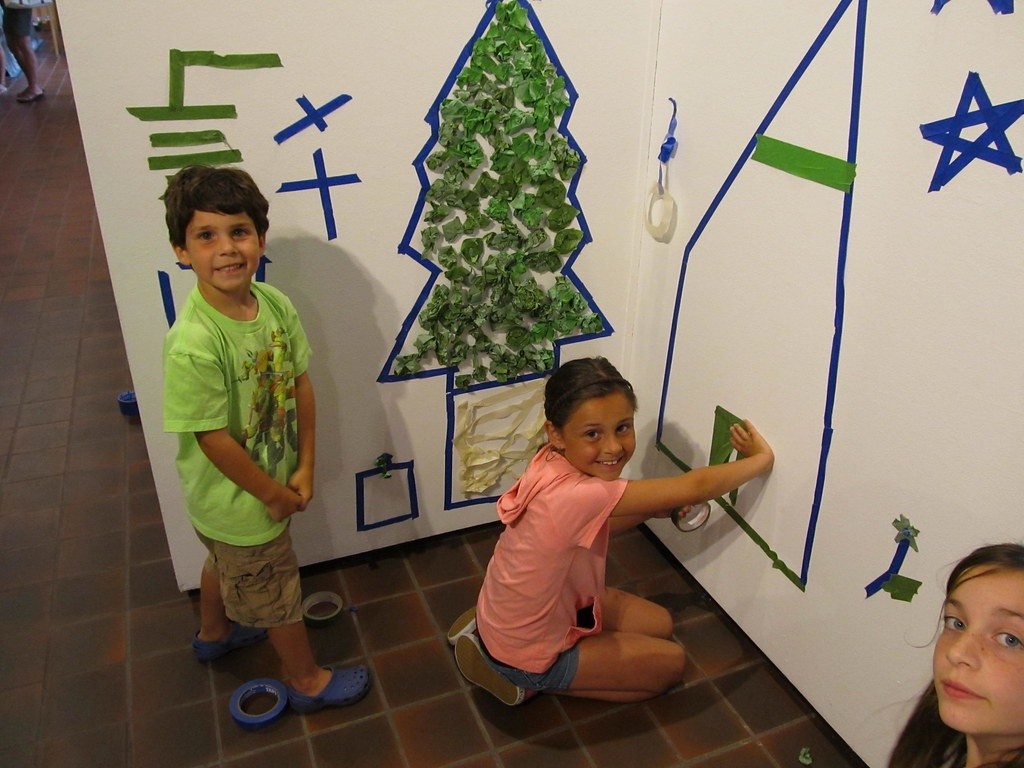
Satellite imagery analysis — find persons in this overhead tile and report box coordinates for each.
[888,543,1024,768]
[447,358,774,705]
[0,0,44,102]
[161,165,369,711]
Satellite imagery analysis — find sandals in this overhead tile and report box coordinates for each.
[287,665,369,712]
[192,621,267,661]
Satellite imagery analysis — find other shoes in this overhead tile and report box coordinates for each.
[16,86,45,102]
[455,634,538,705]
[447,607,481,646]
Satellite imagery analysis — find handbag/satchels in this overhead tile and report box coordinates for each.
[4,0,52,9]
